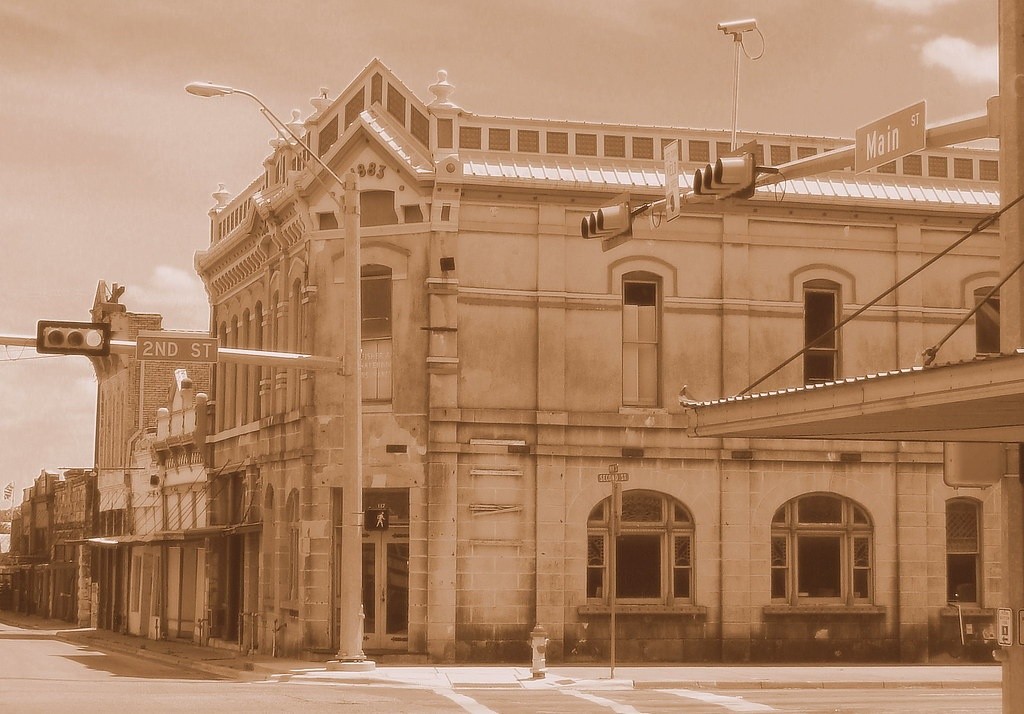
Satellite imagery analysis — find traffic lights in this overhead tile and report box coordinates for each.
[36,318,112,358]
[363,508,390,533]
[692,153,755,202]
[581,191,634,253]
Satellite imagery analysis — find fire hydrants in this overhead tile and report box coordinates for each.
[526,621,551,680]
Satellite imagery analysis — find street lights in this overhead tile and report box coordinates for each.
[187,82,378,671]
